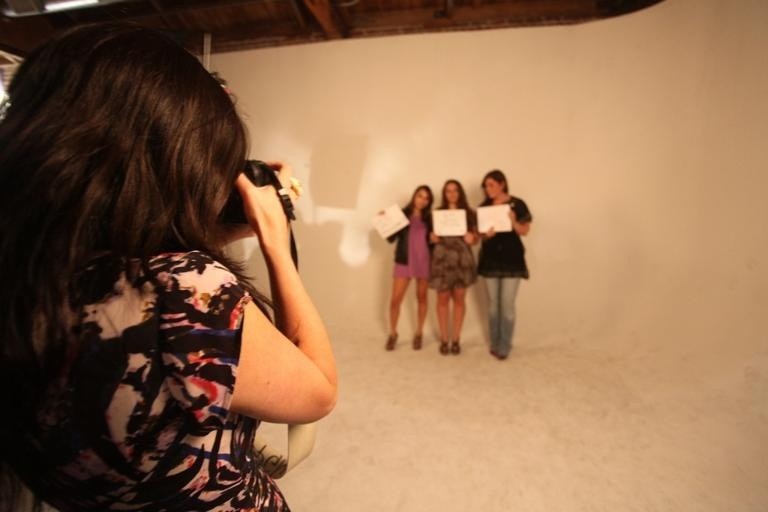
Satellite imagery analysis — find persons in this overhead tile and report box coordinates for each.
[377,185,434,351]
[427,179,479,353]
[477,168,532,361]
[1,17,340,511]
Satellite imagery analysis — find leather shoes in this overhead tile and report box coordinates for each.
[385,333,461,355]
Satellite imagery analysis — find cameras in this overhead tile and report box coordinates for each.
[215,160,296,226]
